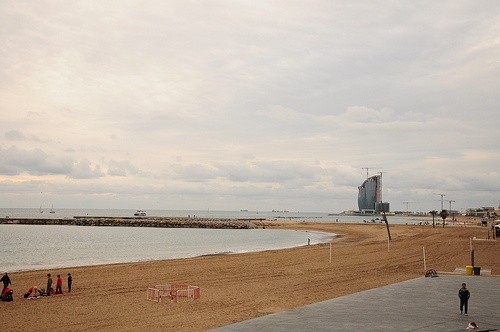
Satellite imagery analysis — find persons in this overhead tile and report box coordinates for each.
[50,287,63,295]
[67,273,73,293]
[45,273,53,296]
[56,274,63,295]
[0,272,11,295]
[458,282,470,316]
[24,286,44,298]
